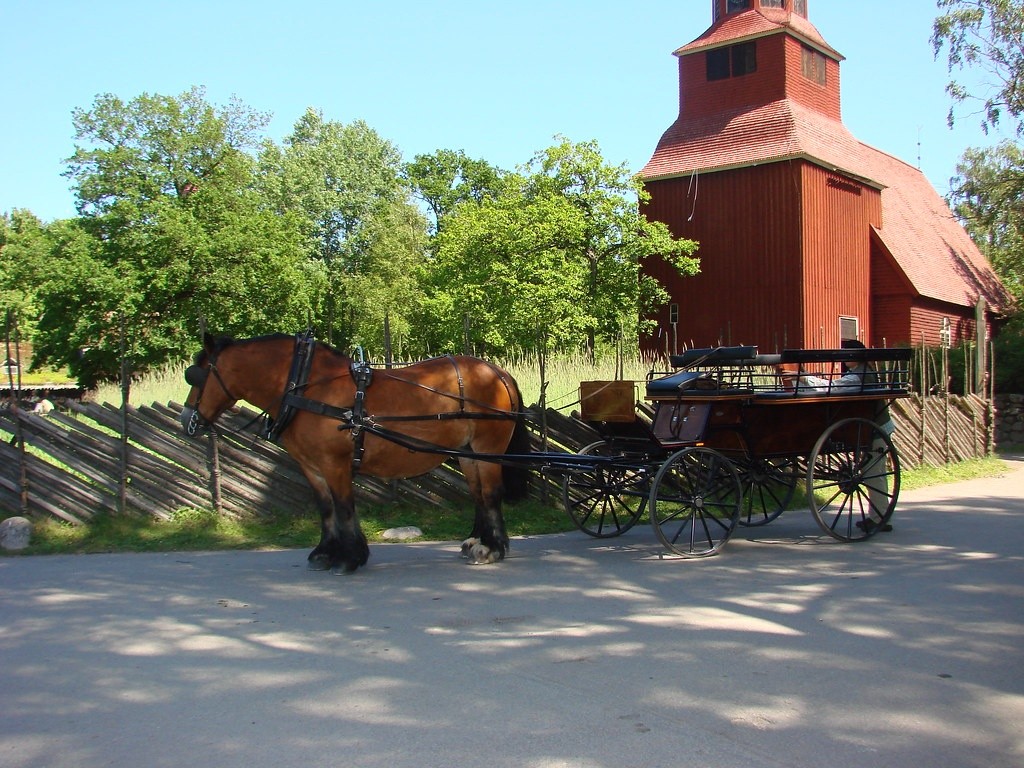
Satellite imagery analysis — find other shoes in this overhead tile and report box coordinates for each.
[856,518,872,528]
[862,523,892,532]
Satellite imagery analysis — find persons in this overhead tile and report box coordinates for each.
[782,340,897,533]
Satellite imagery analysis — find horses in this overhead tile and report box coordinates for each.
[180,307,533,578]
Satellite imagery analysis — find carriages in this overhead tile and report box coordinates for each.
[180,329,918,579]
